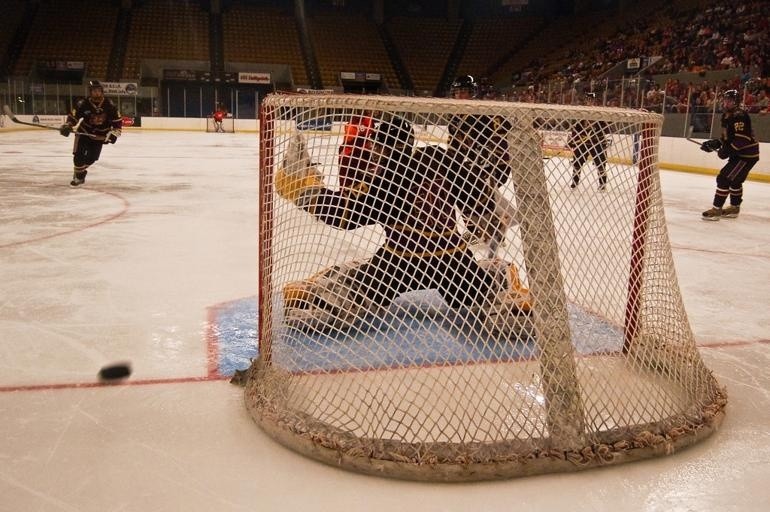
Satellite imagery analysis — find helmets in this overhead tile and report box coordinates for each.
[584,91,597,107]
[369,118,414,161]
[450,75,479,100]
[88,80,103,95]
[722,89,742,112]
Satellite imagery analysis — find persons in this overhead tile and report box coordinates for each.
[60,79,124,186]
[273,115,537,338]
[698,89,761,219]
[565,90,611,192]
[210,106,227,132]
[446,74,512,250]
[340,86,385,193]
[443,1,768,117]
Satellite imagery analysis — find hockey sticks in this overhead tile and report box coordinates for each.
[4,104,113,144]
[686,127,720,153]
[543,144,566,159]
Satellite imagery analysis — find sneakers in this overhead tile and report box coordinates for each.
[703,207,722,216]
[599,183,606,189]
[571,180,578,187]
[71,177,84,186]
[492,228,505,242]
[462,230,481,243]
[722,206,739,214]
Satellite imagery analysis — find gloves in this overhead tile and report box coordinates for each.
[105,130,121,144]
[718,148,730,159]
[61,125,72,136]
[701,139,721,152]
